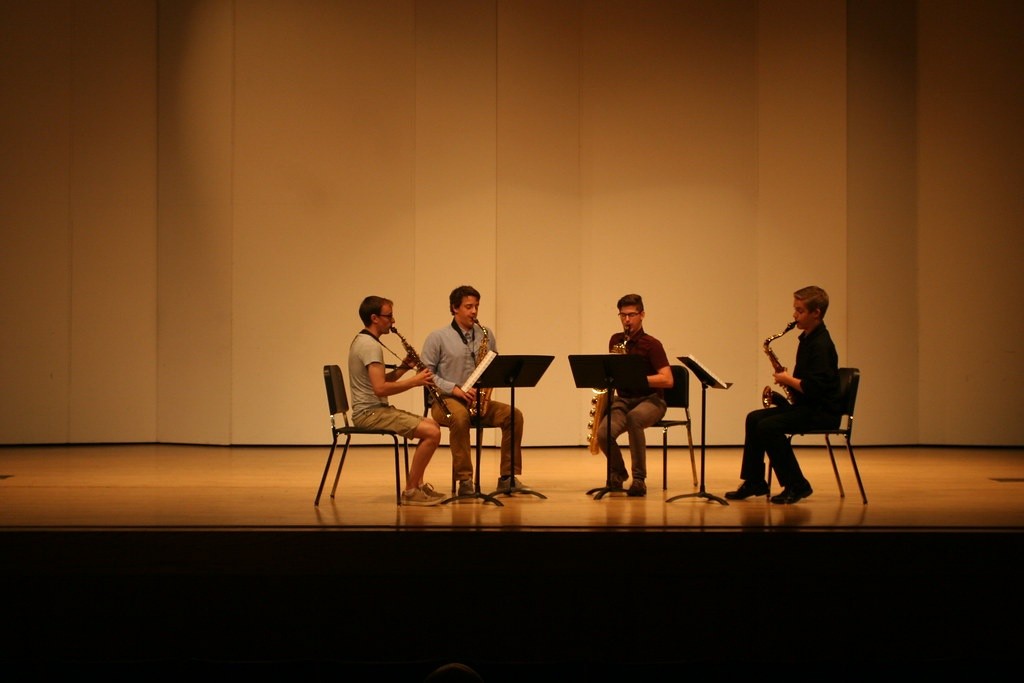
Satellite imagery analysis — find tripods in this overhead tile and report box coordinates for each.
[568,355,655,500]
[665,355,733,506]
[440,351,554,507]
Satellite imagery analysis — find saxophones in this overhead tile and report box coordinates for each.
[762,320,798,408]
[587,323,632,455]
[388,326,453,419]
[468,314,500,420]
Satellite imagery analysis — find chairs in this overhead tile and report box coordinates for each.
[767,367,869,504]
[315,365,409,505]
[423,385,497,494]
[651,365,698,490]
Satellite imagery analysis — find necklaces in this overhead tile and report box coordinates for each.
[452,317,476,358]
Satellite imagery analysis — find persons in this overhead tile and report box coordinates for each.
[347,296,449,506]
[595,292,675,496]
[417,285,532,497]
[724,284,843,505]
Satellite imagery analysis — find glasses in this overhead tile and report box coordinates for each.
[618,312,641,318]
[372,313,392,319]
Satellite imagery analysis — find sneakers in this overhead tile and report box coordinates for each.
[458,479,474,496]
[418,482,446,500]
[497,476,532,491]
[401,487,443,505]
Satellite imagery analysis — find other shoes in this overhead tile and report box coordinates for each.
[608,471,628,488]
[725,478,770,499]
[627,479,646,496]
[772,480,813,504]
